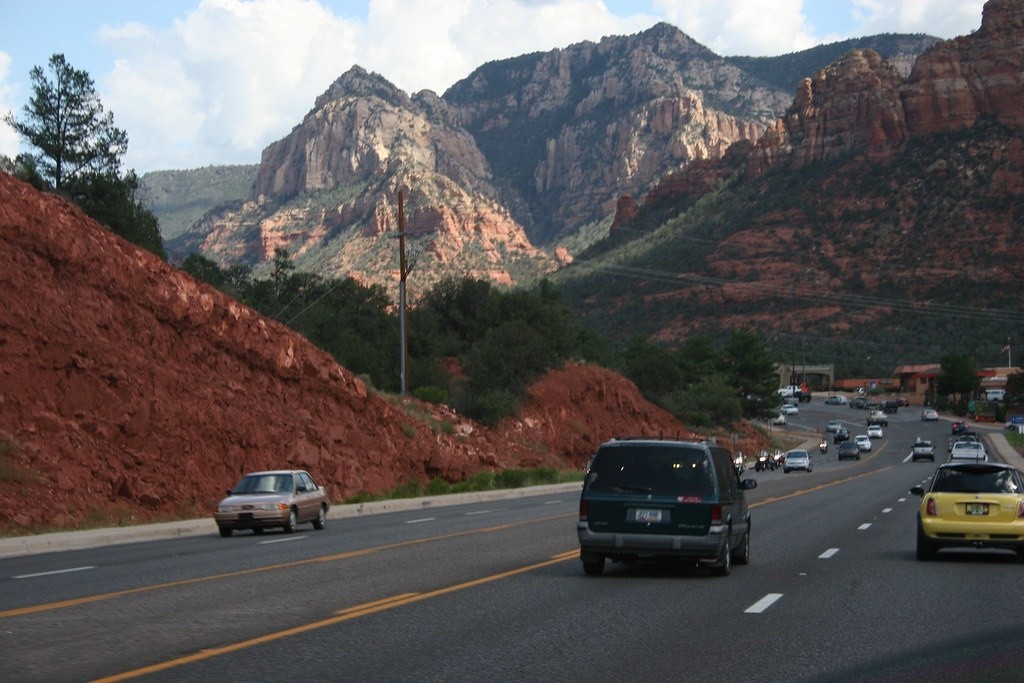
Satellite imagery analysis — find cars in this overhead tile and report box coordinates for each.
[854,435,872,454]
[869,425,883,439]
[782,449,812,475]
[837,441,861,462]
[946,421,989,464]
[910,463,1022,560]
[826,420,850,443]
[213,470,330,537]
[1005,420,1024,431]
[823,395,847,406]
[774,384,813,427]
[922,410,939,422]
[850,397,911,415]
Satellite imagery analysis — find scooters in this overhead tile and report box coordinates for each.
[820,443,828,453]
[734,453,785,473]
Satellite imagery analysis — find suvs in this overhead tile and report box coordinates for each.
[575,437,760,581]
[911,441,937,463]
[868,410,889,426]
